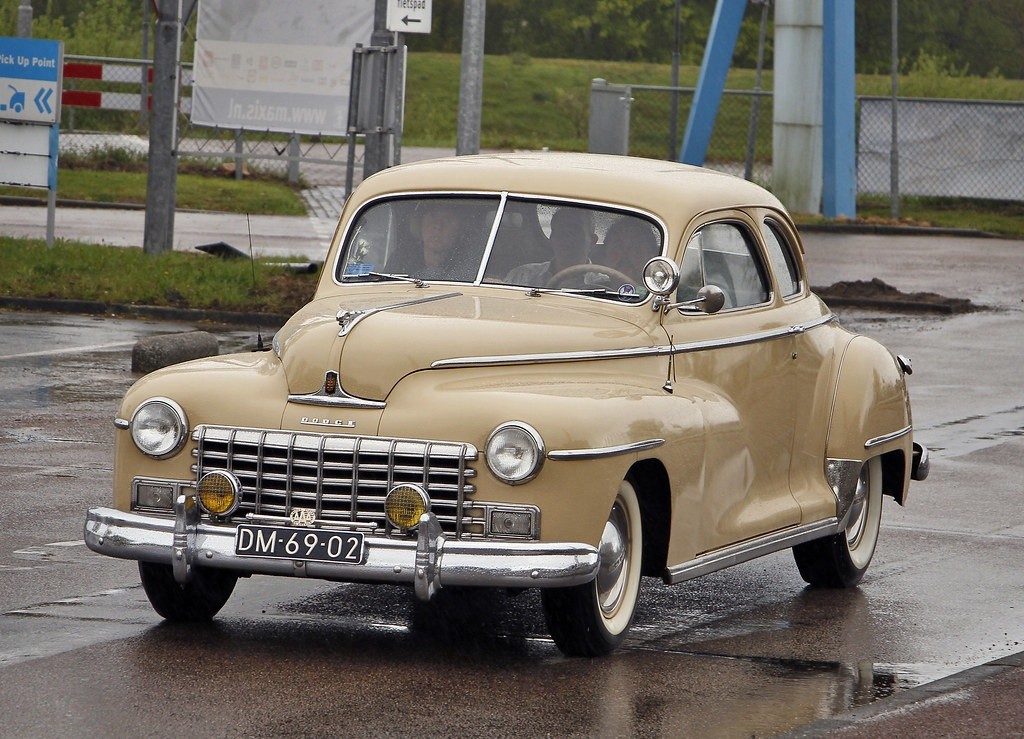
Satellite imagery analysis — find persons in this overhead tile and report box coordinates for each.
[481,209,609,285]
[383,201,480,281]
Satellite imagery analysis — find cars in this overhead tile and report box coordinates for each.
[83,152,932,656]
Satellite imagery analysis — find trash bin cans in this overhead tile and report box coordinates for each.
[587,81,633,156]
